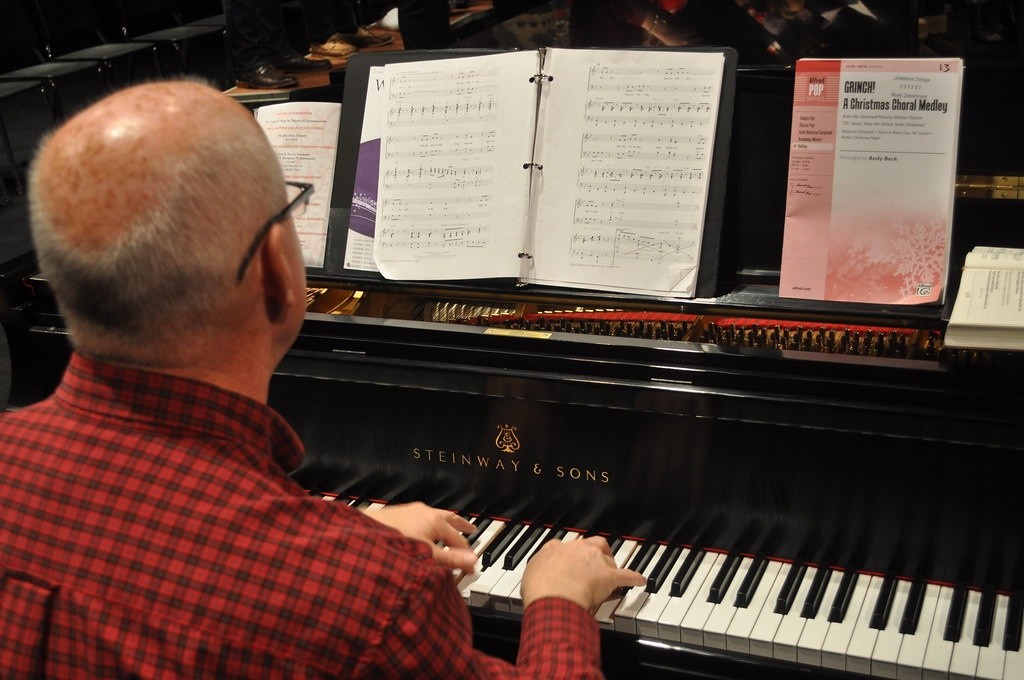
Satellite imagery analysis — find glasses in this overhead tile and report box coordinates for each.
[237,181,314,284]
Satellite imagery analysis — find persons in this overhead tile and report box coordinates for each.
[0,0,1024,63]
[0,77,658,680]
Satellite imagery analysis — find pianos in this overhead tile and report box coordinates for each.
[0,39,1023,680]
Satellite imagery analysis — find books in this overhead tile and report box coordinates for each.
[341,49,728,300]
[251,101,342,269]
[943,247,1024,353]
[776,57,964,308]
